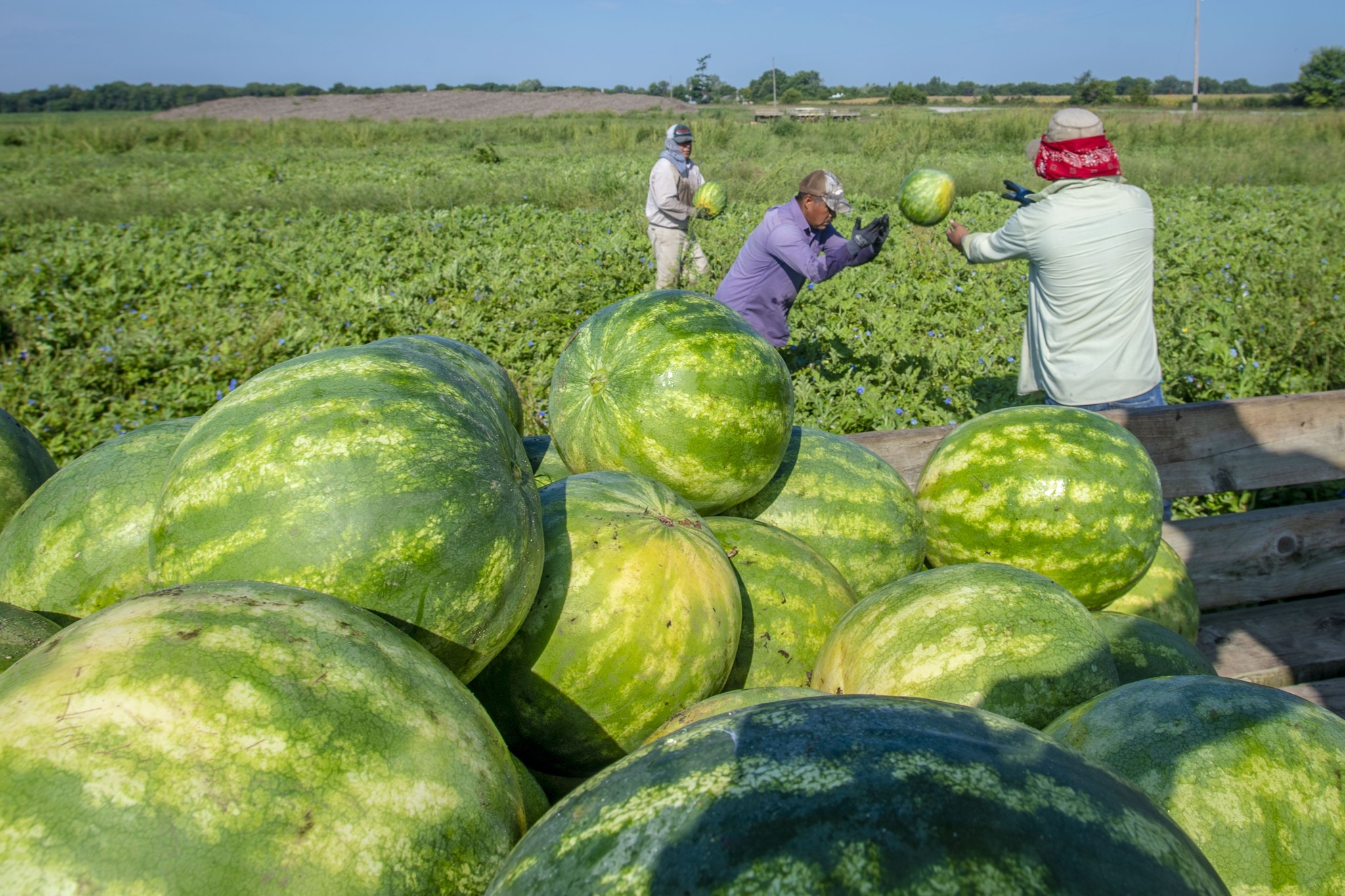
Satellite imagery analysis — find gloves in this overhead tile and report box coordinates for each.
[872,214,889,255]
[695,208,712,221]
[1001,180,1036,207]
[846,217,882,253]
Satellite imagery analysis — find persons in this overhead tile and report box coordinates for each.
[645,123,713,290]
[945,106,1165,412]
[713,170,890,345]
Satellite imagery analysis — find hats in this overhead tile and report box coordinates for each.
[1027,108,1104,167]
[666,124,695,143]
[799,169,853,214]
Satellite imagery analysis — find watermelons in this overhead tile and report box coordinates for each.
[0,290,1345,896]
[897,168,954,227]
[691,181,728,220]
